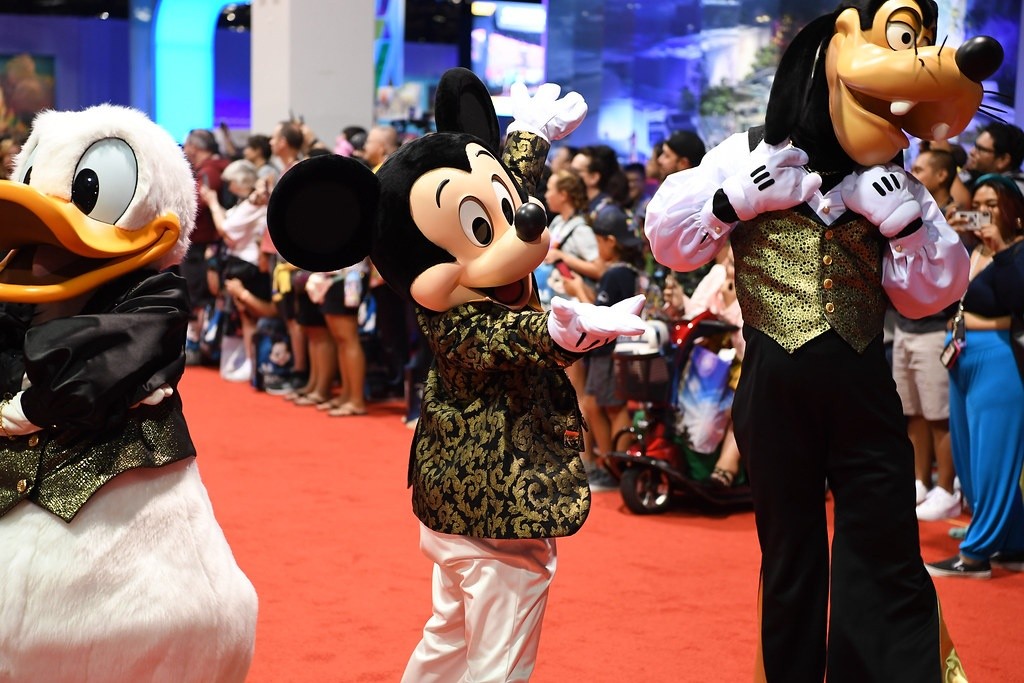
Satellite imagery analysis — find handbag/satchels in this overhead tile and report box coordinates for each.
[534,223,597,304]
[220,295,253,382]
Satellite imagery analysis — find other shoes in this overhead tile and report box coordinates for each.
[702,468,740,498]
[586,462,620,491]
[949,525,969,538]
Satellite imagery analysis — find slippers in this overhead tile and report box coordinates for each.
[295,394,326,406]
[317,399,340,411]
[329,402,366,416]
[285,389,305,400]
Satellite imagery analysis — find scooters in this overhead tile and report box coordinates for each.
[611,317,755,514]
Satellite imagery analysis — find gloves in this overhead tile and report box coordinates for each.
[841,164,923,238]
[722,138,822,221]
[548,295,646,353]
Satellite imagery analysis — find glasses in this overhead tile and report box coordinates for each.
[974,141,994,153]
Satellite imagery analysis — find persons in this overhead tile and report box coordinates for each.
[0,107,1024,583]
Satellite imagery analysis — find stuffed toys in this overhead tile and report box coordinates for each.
[1,101,258,683]
[266,66,649,683]
[640,0,1006,683]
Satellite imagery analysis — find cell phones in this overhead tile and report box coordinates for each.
[954,209,992,231]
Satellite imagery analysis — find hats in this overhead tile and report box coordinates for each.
[593,204,640,247]
[666,130,706,164]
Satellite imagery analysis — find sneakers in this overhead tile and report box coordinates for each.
[923,555,993,577]
[991,554,1024,571]
[915,480,961,521]
